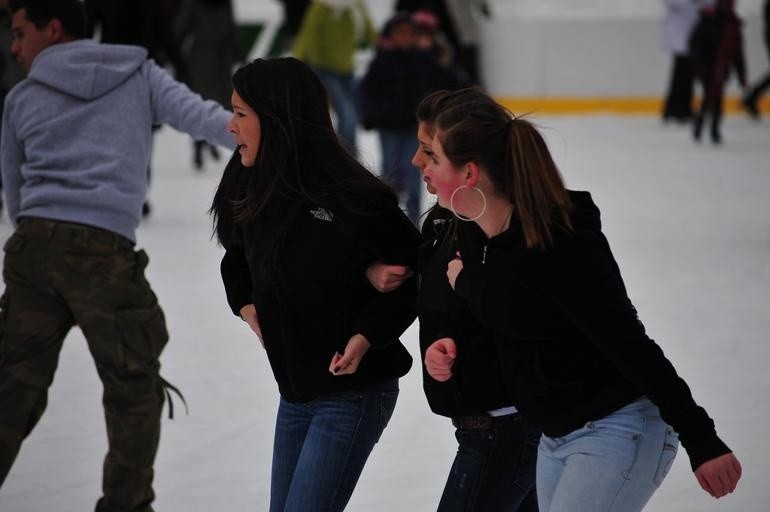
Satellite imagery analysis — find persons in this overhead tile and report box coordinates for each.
[687,1,750,144]
[363,90,542,510]
[424,92,742,511]
[661,0,707,122]
[1,0,243,510]
[737,0,770,121]
[207,58,422,509]
